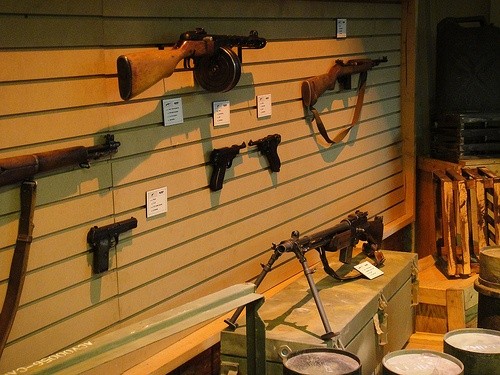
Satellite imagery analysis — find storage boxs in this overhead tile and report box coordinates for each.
[415,252,480,336]
[220,248,421,375]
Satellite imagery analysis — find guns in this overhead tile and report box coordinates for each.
[248,133,281,173]
[301,57,388,144]
[87,217,137,274]
[0,132,122,356]
[117,27,268,101]
[211,142,247,192]
[223,207,384,340]
